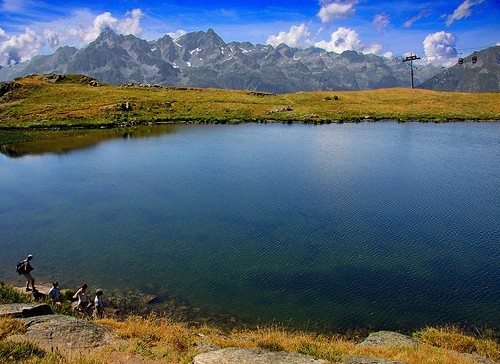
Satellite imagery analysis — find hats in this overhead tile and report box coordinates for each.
[28,255,33,259]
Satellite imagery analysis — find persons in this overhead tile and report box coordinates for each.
[73,284,90,310]
[94,289,105,319]
[48,281,61,305]
[23,255,39,292]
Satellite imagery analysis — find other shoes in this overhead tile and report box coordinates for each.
[33,289,39,292]
[26,288,32,292]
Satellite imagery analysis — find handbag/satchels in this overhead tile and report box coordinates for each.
[16,260,27,275]
